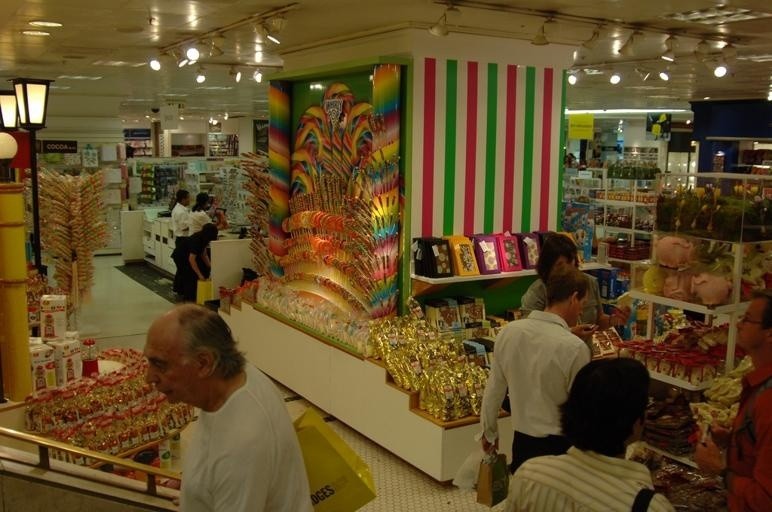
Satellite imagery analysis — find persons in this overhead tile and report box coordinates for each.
[562,153,588,171]
[143,305,316,512]
[690,290,772,511]
[469,264,595,477]
[506,355,677,512]
[170,222,218,302]
[170,188,192,298]
[518,232,633,344]
[189,193,224,267]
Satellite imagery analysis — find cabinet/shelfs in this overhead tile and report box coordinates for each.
[142,218,177,279]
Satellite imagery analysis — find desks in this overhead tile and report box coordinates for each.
[561,164,659,309]
[628,172,772,392]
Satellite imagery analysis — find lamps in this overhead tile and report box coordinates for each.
[264,13,285,45]
[148,35,244,88]
[428,3,461,38]
[529,17,738,87]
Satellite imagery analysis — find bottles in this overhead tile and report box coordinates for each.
[606,159,662,191]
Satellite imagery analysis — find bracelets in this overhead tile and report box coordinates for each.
[608,314,612,325]
[719,468,729,479]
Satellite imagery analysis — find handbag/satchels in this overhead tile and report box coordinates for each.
[293,408,376,512]
[477,453,513,507]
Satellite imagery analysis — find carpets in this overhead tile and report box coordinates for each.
[113,264,220,314]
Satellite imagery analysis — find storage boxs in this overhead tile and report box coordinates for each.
[27,294,84,390]
